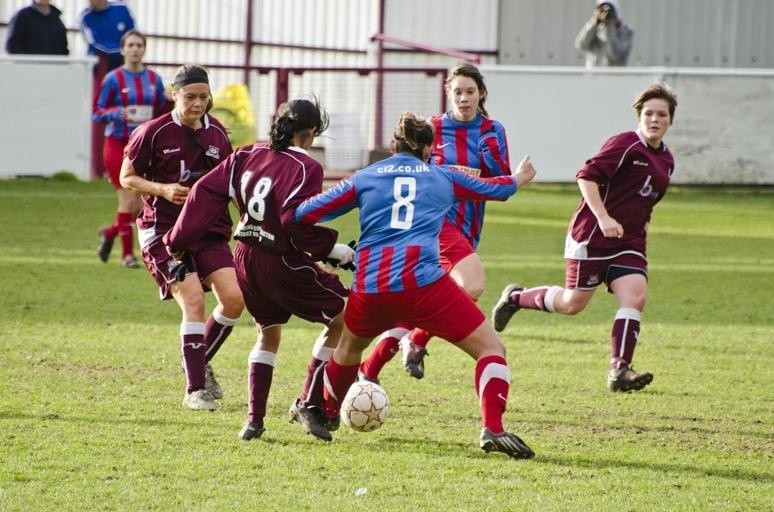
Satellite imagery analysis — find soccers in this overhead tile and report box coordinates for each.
[340,382,389,432]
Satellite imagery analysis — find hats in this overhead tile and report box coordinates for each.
[171,64,208,86]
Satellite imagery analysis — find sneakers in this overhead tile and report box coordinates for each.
[357,361,379,386]
[119,254,140,268]
[182,388,220,411]
[97,226,114,262]
[239,418,266,440]
[288,399,340,440]
[181,360,223,400]
[398,330,429,378]
[607,365,654,392]
[480,427,535,459]
[492,284,525,333]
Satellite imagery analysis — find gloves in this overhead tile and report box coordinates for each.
[168,246,193,281]
[323,241,357,271]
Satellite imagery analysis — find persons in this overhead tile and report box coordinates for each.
[119,64,245,411]
[162,91,356,441]
[356,64,511,386]
[492,85,677,391]
[293,111,536,460]
[574,0,634,67]
[90,30,165,268]
[5,1,69,54]
[79,0,138,69]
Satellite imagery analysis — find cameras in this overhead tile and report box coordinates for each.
[599,6,614,18]
